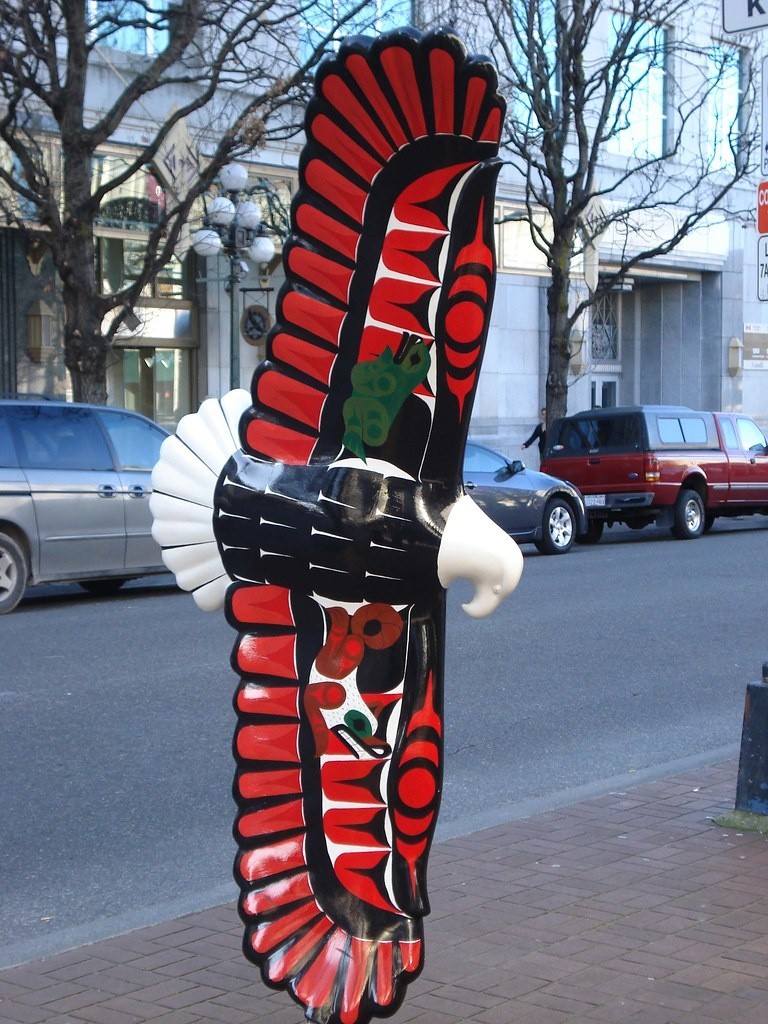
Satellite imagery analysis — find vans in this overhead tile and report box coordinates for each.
[540,405,768,543]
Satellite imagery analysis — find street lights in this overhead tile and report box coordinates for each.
[193,163,275,389]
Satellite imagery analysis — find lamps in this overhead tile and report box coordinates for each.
[25,299,54,364]
[728,337,745,376]
[572,330,587,377]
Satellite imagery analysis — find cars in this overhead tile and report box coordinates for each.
[462,440,587,555]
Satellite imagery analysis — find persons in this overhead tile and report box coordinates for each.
[522,409,546,462]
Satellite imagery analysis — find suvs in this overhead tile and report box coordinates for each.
[0,393,173,614]
